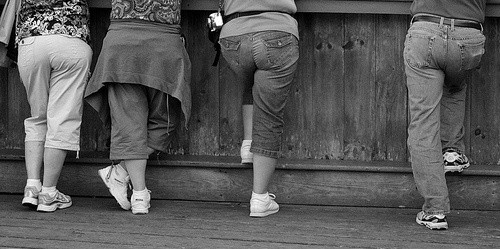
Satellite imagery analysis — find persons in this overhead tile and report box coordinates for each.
[404,1,485,229]
[85,0,191,214]
[15,0,93,211]
[219,0,299,217]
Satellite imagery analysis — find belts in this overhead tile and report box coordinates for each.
[412,16,481,30]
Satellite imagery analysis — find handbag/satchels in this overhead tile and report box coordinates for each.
[207,10,224,67]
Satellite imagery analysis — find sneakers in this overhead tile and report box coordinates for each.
[98,161,131,210]
[22,182,42,206]
[36,189,72,212]
[240,145,254,163]
[415,210,448,230]
[443,148,470,174]
[131,189,152,214]
[249,191,280,217]
